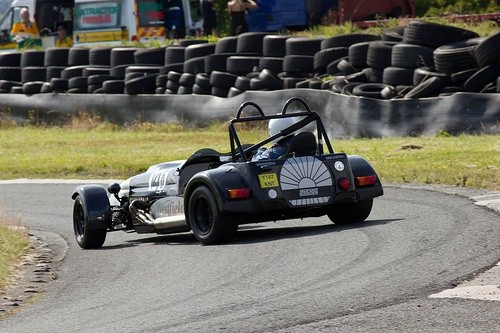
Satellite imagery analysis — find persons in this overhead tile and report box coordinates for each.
[162,0,186,40]
[9,7,40,48]
[49,3,72,33]
[56,26,73,47]
[201,0,218,36]
[251,113,297,161]
[228,0,257,36]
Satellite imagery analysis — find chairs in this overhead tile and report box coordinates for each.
[278,131,317,158]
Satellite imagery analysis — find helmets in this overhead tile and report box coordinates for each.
[268,113,296,142]
